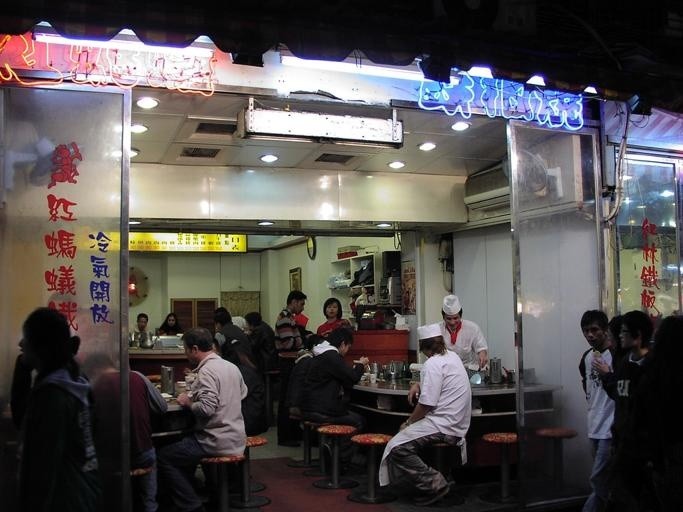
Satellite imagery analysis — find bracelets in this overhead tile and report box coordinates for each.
[406,419,409,426]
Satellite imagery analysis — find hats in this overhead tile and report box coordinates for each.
[417,323,443,340]
[219,337,241,353]
[442,294,462,315]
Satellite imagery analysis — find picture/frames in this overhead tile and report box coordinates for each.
[287,266,302,291]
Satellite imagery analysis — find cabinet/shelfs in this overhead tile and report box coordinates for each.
[326,249,382,315]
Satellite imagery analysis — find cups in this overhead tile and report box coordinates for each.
[361,373,376,383]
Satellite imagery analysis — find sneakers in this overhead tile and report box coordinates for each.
[413,484,449,506]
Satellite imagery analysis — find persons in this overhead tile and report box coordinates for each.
[440,295,490,372]
[84,353,169,512]
[579,311,683,512]
[129,313,154,341]
[214,290,370,478]
[157,326,248,512]
[10,308,105,512]
[379,324,472,506]
[159,313,184,336]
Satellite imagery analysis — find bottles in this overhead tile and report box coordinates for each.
[140,329,157,348]
[162,366,174,395]
[177,380,187,394]
[489,357,502,384]
[370,359,409,379]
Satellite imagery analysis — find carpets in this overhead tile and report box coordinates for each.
[242,456,391,510]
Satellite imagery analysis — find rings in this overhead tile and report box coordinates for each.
[416,393,419,395]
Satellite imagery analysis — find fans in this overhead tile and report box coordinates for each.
[499,146,565,205]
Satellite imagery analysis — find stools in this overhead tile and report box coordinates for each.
[428,441,450,475]
[117,373,272,511]
[287,415,394,505]
[535,426,579,504]
[483,433,527,506]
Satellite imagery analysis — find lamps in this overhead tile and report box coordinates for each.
[226,48,266,69]
[417,55,451,87]
[626,91,655,116]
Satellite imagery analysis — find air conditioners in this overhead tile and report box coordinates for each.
[461,168,543,214]
